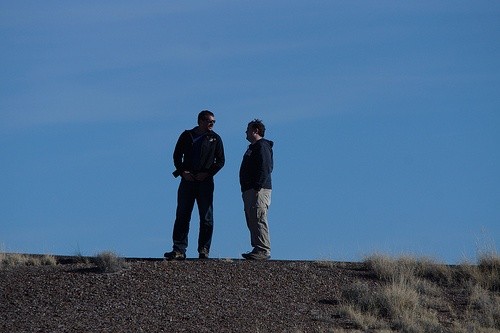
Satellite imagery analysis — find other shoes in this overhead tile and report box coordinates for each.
[242,251,271,260]
[199,250,209,258]
[164,250,186,258]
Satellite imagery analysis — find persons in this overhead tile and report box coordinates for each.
[164,110,225,259]
[239,119,274,260]
